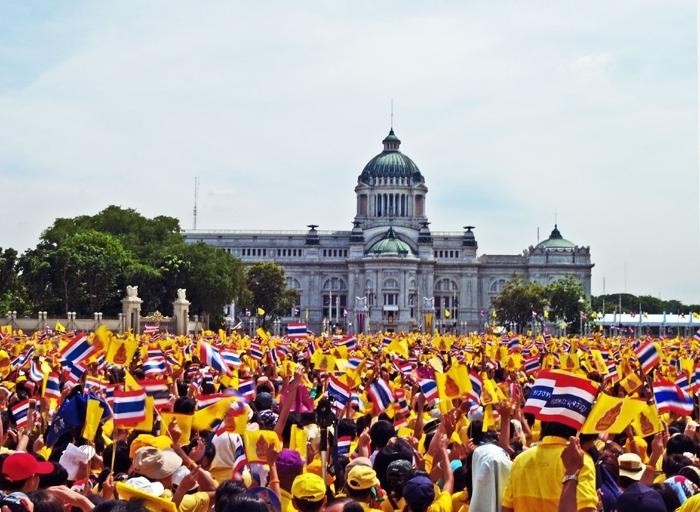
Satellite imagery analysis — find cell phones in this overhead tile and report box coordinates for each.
[28,399,36,411]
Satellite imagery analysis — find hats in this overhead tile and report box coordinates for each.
[274,449,307,474]
[617,451,648,482]
[170,463,200,493]
[256,409,280,430]
[289,472,328,502]
[345,464,381,490]
[131,445,183,480]
[385,458,417,479]
[0,451,56,483]
[400,474,435,506]
[422,417,443,436]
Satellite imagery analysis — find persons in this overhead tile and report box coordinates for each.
[0,310,700,512]
